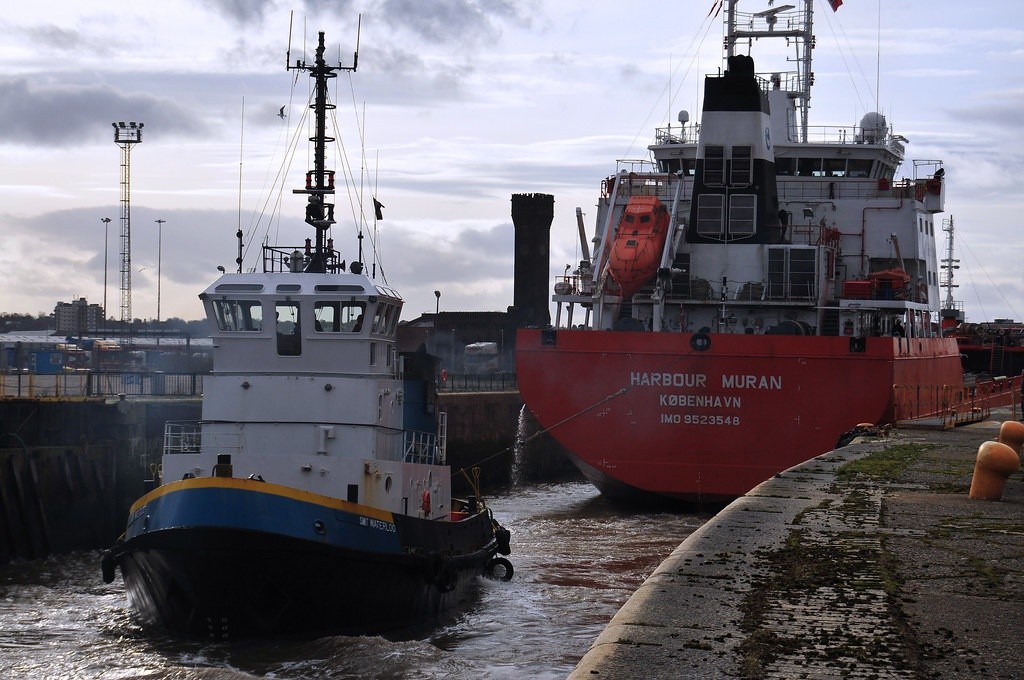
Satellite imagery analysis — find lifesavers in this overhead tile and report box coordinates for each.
[691,333,709,351]
[422,490,432,512]
[441,368,448,382]
[488,557,514,582]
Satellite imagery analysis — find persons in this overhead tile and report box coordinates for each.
[892,319,905,338]
[934,168,944,179]
[352,315,362,332]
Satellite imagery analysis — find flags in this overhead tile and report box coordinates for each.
[373,198,385,220]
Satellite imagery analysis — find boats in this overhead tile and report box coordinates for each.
[115,11,500,643]
[931,215,1024,374]
[511,0,970,502]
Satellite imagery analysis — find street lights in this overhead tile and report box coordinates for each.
[155,219,167,329]
[102,218,113,339]
[434,291,441,315]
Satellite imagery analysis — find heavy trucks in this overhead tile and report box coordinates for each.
[463,342,514,380]
